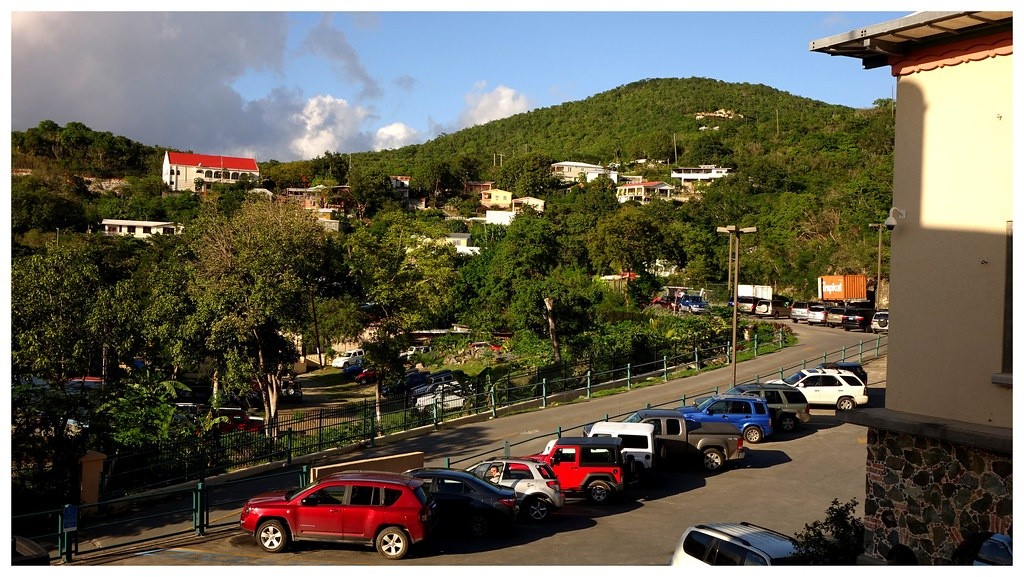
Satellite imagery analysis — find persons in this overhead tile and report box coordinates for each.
[489,466,500,483]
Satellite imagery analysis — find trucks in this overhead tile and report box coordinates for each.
[171,401,265,436]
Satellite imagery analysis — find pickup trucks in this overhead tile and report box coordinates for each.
[582,409,745,476]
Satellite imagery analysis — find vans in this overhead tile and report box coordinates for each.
[788,301,820,324]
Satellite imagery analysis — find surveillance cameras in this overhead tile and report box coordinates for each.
[885,217,897,230]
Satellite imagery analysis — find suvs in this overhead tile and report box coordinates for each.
[506,435,627,507]
[540,422,668,480]
[737,297,759,315]
[281,378,303,401]
[668,520,808,565]
[673,362,873,443]
[753,299,792,319]
[239,469,442,562]
[870,312,889,334]
[843,305,877,333]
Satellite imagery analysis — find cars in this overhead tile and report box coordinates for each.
[826,307,846,328]
[808,305,830,326]
[726,296,738,308]
[330,341,503,416]
[653,295,711,314]
[389,466,519,542]
[444,455,566,525]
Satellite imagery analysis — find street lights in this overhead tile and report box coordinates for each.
[868,222,888,311]
[715,224,760,388]
